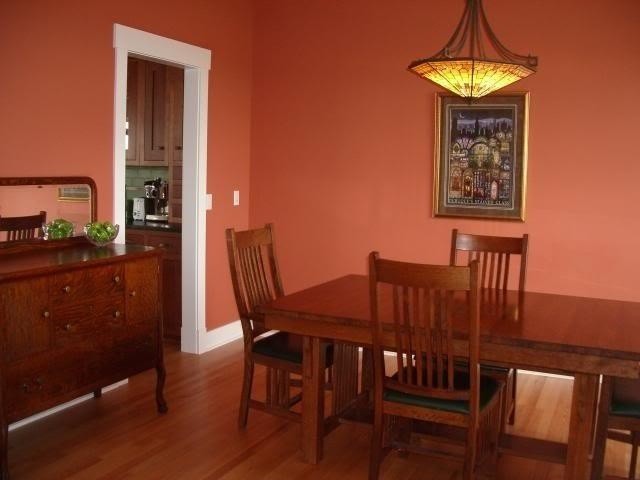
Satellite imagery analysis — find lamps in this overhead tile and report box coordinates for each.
[405,0,541,107]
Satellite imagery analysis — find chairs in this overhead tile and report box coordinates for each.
[364,245,505,478]
[223,219,365,467]
[586,371,639,479]
[412,227,529,428]
[0,209,47,246]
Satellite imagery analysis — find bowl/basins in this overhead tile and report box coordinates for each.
[82,224,120,248]
[41,221,77,242]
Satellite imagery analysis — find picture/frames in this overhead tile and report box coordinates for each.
[430,88,532,224]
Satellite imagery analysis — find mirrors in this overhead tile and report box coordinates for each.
[1,176,99,250]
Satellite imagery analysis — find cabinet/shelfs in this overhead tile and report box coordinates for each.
[166,160,183,229]
[149,228,183,344]
[138,60,171,170]
[123,226,148,247]
[164,62,185,169]
[125,56,141,171]
[0,242,173,479]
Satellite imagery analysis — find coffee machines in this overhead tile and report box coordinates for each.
[144,177,169,222]
